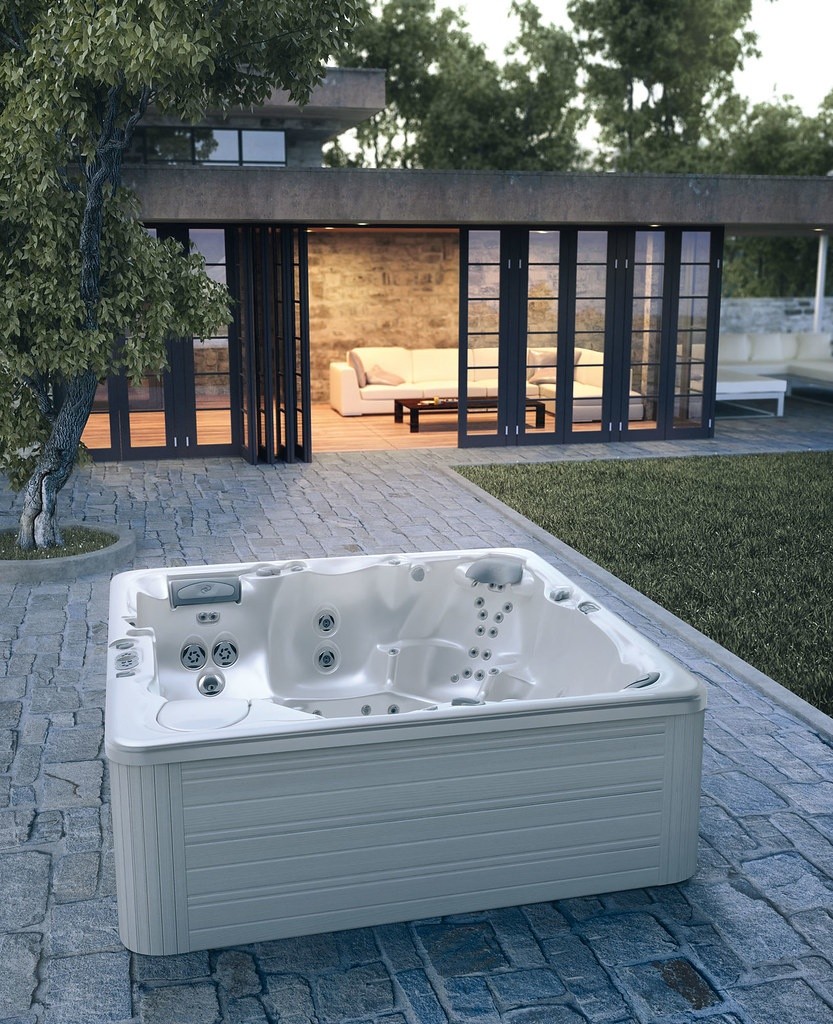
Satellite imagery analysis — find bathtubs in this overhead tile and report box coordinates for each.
[102,546,710,956]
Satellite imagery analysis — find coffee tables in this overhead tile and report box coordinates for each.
[686,369,788,417]
[395,397,545,433]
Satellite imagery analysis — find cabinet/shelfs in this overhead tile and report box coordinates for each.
[88,372,164,412]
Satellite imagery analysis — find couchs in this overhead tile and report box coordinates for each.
[329,346,646,423]
[676,331,832,407]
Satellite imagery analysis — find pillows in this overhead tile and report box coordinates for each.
[528,367,557,384]
[750,333,798,361]
[717,332,751,362]
[410,349,475,382]
[354,348,412,384]
[349,349,368,388]
[365,366,406,385]
[529,351,582,384]
[474,348,499,379]
[796,333,832,361]
